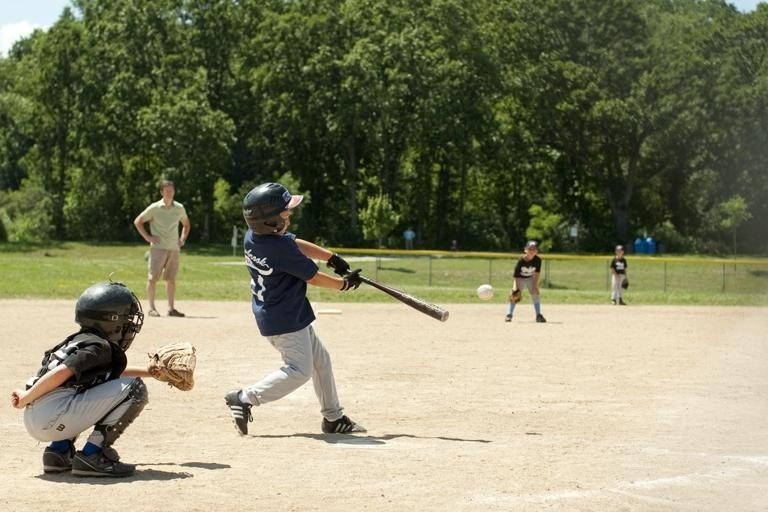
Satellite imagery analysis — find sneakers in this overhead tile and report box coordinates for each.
[43,446,76,471]
[225,390,252,436]
[148,310,160,317]
[536,314,546,322]
[321,415,368,433]
[72,446,136,476]
[168,310,184,317]
[505,314,512,322]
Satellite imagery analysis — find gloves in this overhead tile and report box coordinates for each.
[340,268,362,291]
[327,253,349,275]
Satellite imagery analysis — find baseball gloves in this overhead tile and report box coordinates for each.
[508,288,520,303]
[622,277,628,289]
[147,343,196,391]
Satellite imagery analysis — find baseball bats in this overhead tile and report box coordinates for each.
[346,269,449,322]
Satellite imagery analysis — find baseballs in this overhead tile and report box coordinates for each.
[477,284,494,300]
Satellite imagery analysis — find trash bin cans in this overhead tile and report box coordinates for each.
[635,237,656,254]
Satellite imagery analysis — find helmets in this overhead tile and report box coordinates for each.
[75,281,144,350]
[242,183,303,233]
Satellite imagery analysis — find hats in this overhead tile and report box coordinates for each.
[526,240,538,250]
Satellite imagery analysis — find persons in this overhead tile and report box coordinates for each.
[646,232,658,253]
[10,280,193,477]
[632,232,646,254]
[223,183,370,434]
[504,240,548,322]
[403,223,418,249]
[451,237,460,250]
[610,245,627,307]
[133,180,191,319]
